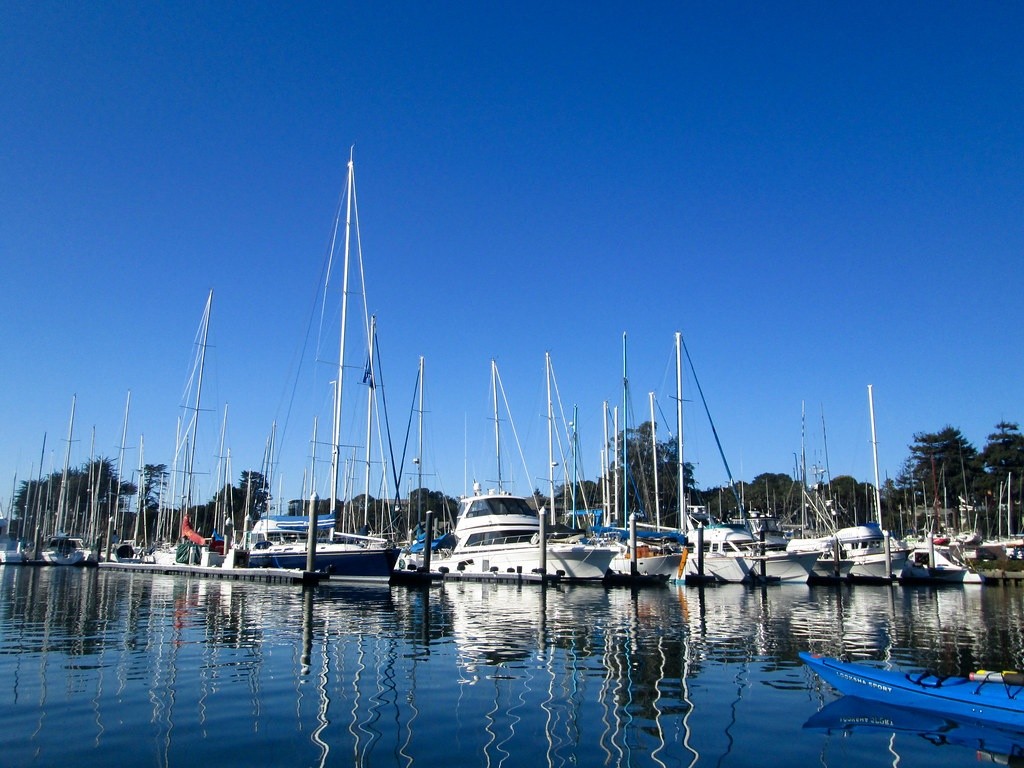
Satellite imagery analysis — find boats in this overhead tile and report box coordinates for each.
[801,696,1024,768]
[798,650,1024,735]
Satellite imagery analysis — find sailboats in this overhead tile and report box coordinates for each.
[0,136,1024,584]
